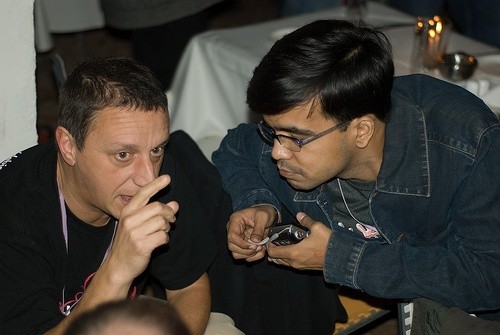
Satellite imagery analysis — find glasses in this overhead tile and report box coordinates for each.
[256,119,353,152]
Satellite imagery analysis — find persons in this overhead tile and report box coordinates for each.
[212,17,500,323]
[63,297,194,335]
[0,56,222,335]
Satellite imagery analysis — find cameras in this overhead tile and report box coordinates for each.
[268,225,310,246]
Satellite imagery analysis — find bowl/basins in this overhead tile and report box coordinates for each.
[436,51,478,82]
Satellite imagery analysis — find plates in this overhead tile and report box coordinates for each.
[474,52,500,84]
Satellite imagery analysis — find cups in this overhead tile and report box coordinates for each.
[411,17,450,69]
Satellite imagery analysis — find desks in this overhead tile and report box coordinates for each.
[165,3,500,165]
